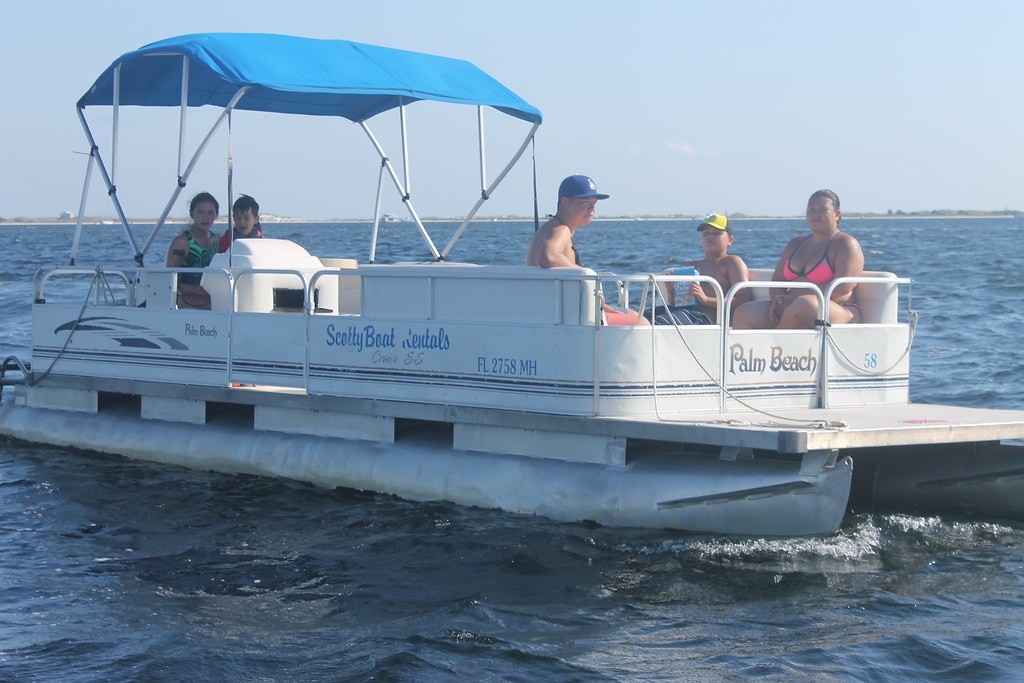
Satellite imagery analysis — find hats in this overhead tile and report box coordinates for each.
[559,174,609,199]
[697,214,733,236]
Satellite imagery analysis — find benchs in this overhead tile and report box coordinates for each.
[658,267,899,322]
[338,264,605,324]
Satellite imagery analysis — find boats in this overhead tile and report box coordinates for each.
[0,33,1024,538]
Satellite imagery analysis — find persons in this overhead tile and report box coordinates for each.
[525,174,864,330]
[166,191,264,310]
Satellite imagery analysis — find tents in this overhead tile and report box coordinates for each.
[75,31,542,266]
